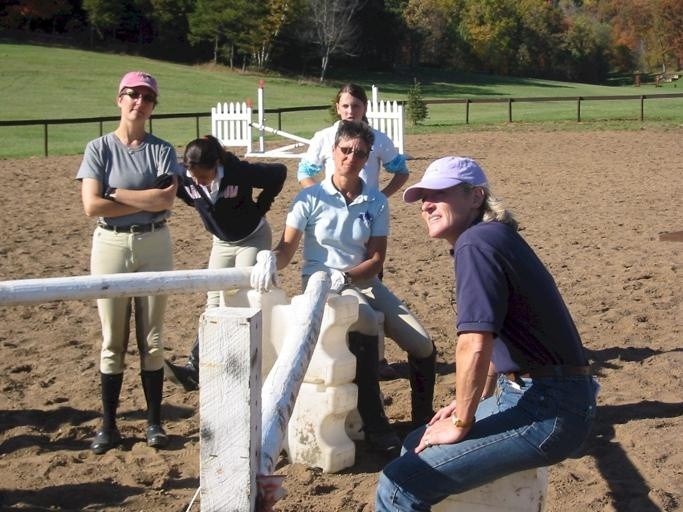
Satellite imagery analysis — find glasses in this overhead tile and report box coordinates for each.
[341,147,366,160]
[120,90,155,102]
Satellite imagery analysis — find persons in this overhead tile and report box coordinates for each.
[75,71,180,454]
[249,119,439,467]
[162,135,286,392]
[297,83,409,200]
[374,156,600,512]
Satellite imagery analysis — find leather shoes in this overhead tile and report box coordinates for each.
[163,358,198,391]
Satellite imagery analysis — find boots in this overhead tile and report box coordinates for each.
[141,367,165,447]
[348,330,402,456]
[407,340,437,426]
[91,372,123,454]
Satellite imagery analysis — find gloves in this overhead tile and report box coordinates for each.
[250,249,279,293]
[153,174,173,189]
[102,186,116,202]
[324,267,352,293]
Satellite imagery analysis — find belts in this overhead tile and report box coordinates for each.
[100,220,166,233]
[505,365,592,386]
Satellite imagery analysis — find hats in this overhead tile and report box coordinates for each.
[117,71,158,95]
[402,156,487,204]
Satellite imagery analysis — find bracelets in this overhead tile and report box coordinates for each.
[104,186,114,199]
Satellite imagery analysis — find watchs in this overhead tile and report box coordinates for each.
[342,270,352,285]
[451,412,477,428]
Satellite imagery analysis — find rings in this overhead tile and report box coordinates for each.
[424,440,431,446]
